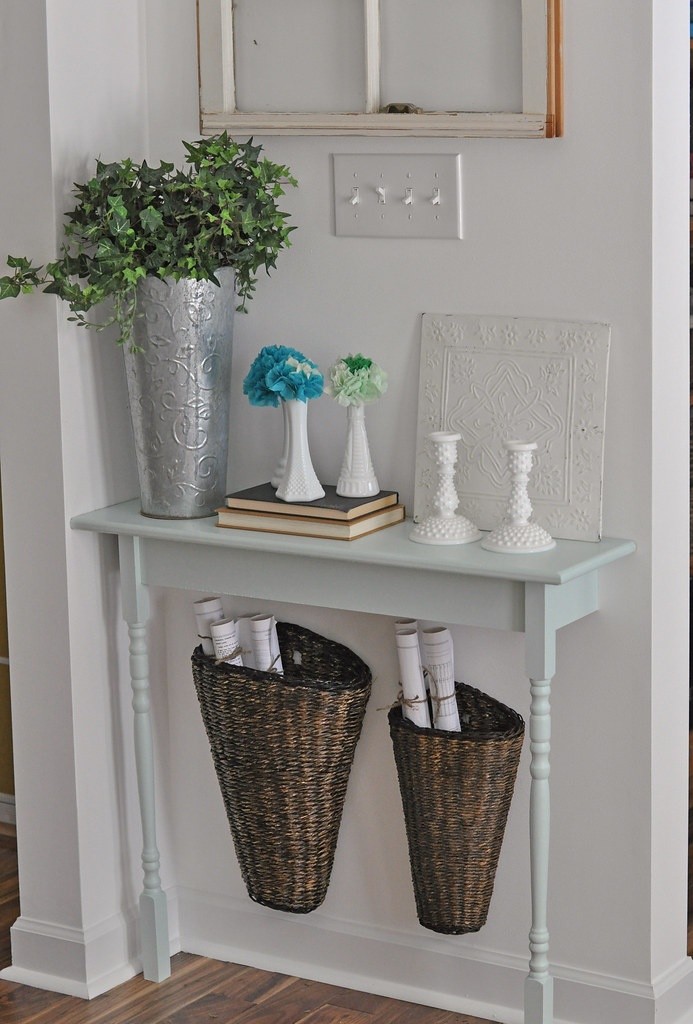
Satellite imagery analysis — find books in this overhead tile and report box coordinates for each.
[215,505,406,541]
[224,482,398,522]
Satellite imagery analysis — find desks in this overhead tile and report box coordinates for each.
[66,495,635,1024]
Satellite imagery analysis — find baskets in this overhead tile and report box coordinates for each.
[191,622,373,915]
[390,679,526,937]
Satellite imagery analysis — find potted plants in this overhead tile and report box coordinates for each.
[0,128,298,521]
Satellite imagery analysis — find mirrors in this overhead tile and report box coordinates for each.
[229,1,527,116]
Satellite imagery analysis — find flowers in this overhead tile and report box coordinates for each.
[242,344,324,408]
[324,353,386,406]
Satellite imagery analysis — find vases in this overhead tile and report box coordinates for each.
[268,396,325,503]
[336,404,379,498]
